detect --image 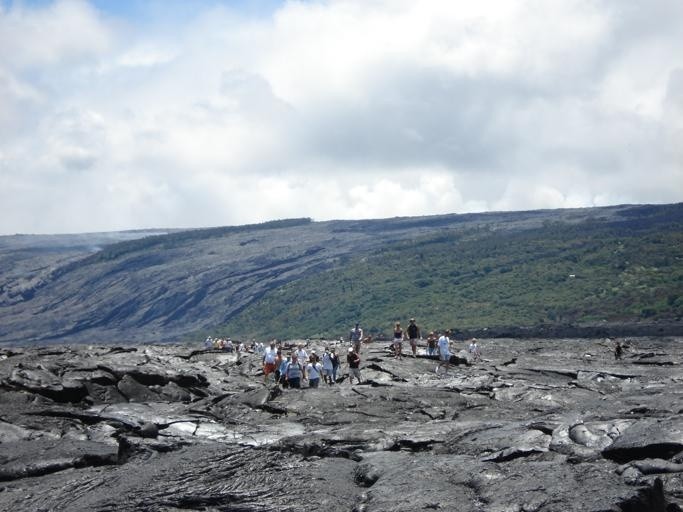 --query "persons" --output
[433,329,451,374]
[425,332,434,358]
[613,341,624,361]
[468,338,478,362]
[434,333,441,354]
[202,323,364,389]
[391,321,404,360]
[405,317,422,357]
